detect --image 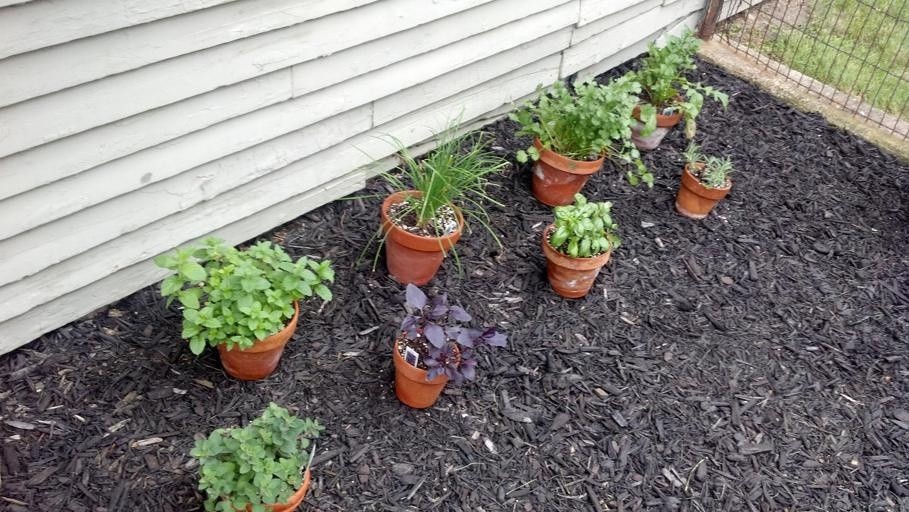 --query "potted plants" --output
[189,401,327,512]
[392,282,509,410]
[511,77,656,207]
[153,236,337,385]
[352,105,507,285]
[675,140,733,222]
[541,191,621,300]
[622,26,730,152]
